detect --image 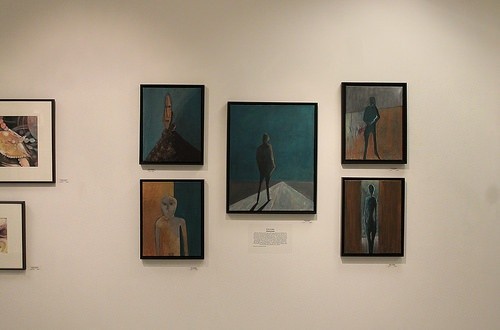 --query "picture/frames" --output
[340,176,405,257]
[139,84,204,166]
[0,201,26,270]
[225,100,317,214]
[0,99,57,184]
[139,178,204,259]
[340,81,408,164]
